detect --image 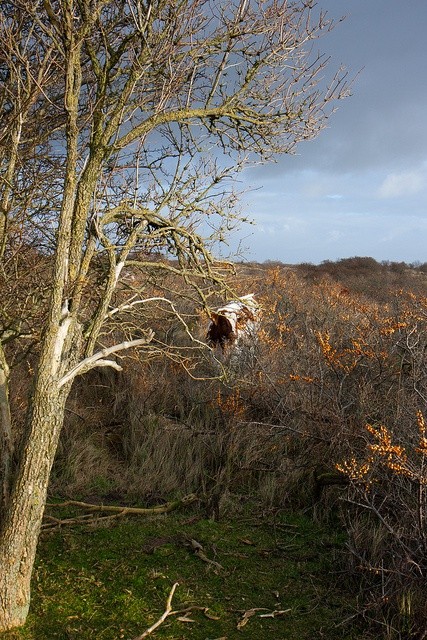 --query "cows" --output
[208,294,266,365]
